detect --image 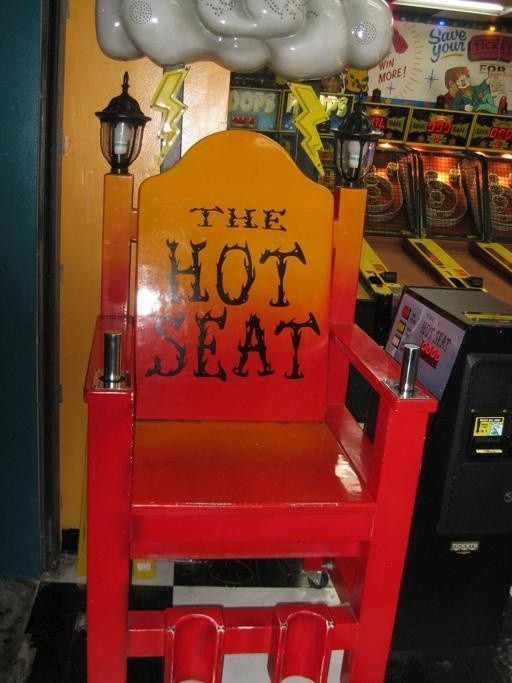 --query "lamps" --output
[94,71,153,176]
[332,106,384,189]
